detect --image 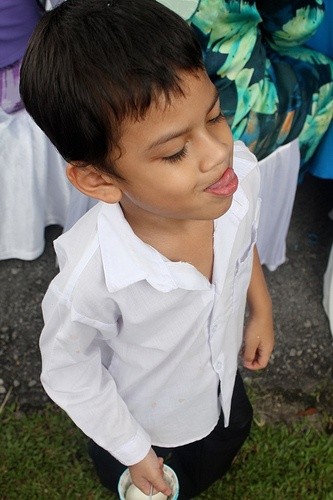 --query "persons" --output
[19,3,274,500]
